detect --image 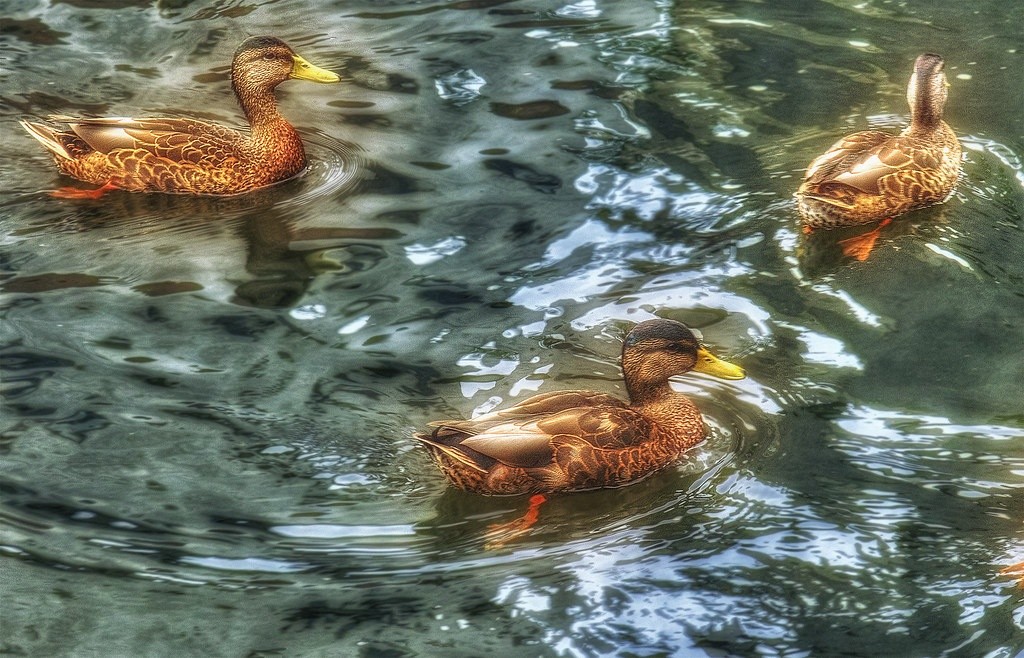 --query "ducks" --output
[19,35,341,199]
[795,52,962,264]
[411,318,747,548]
